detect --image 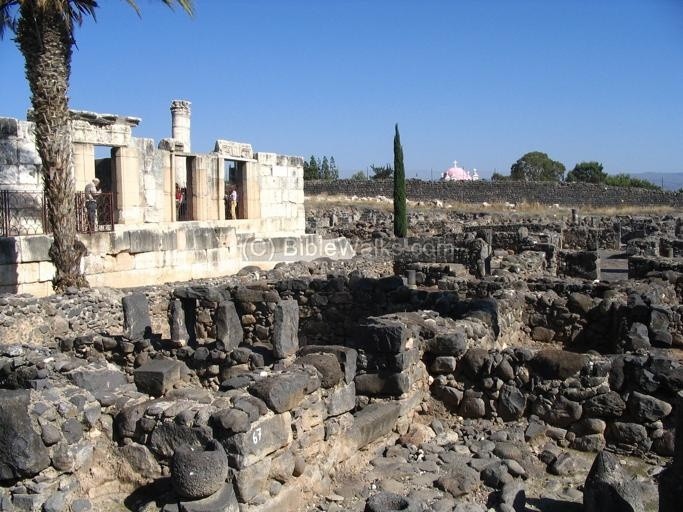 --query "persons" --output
[84,178,102,233]
[176,183,183,220]
[224,184,238,220]
